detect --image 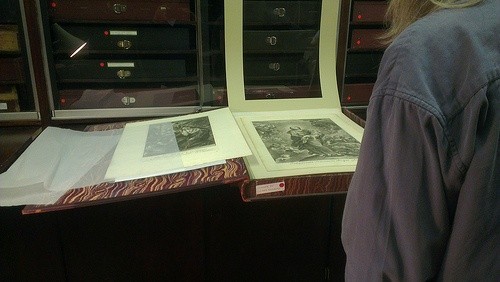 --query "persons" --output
[341,0,500,282]
